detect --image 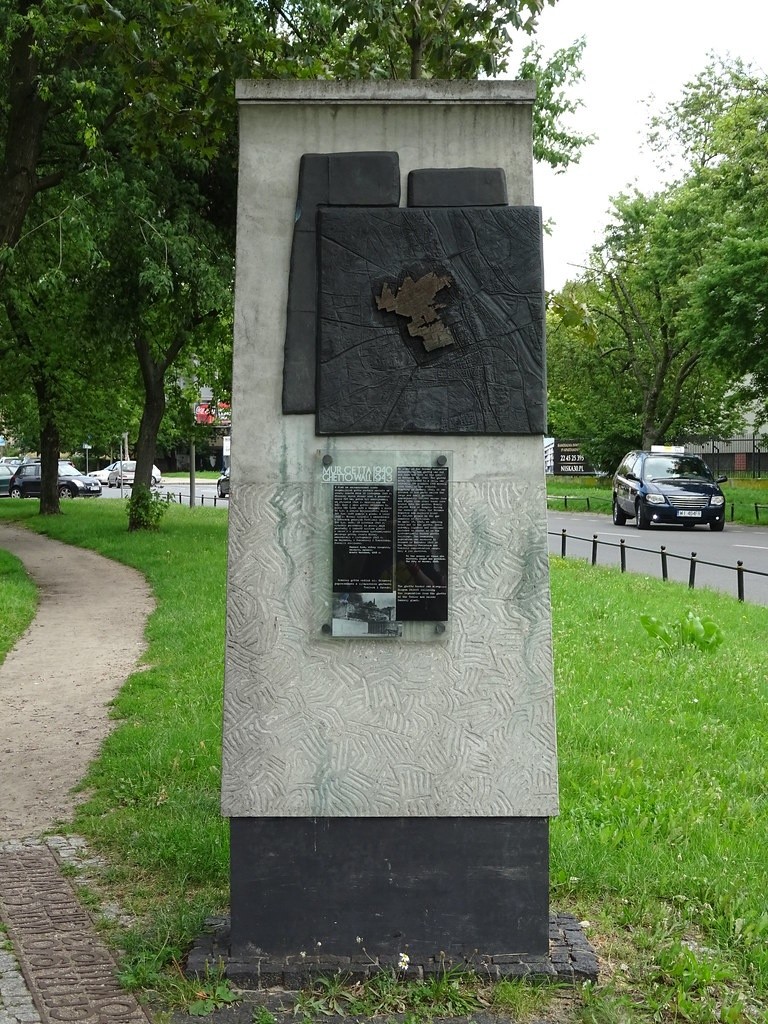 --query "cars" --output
[0,457,102,499]
[88,461,161,486]
[217,466,231,498]
[612,450,728,532]
[107,461,137,488]
